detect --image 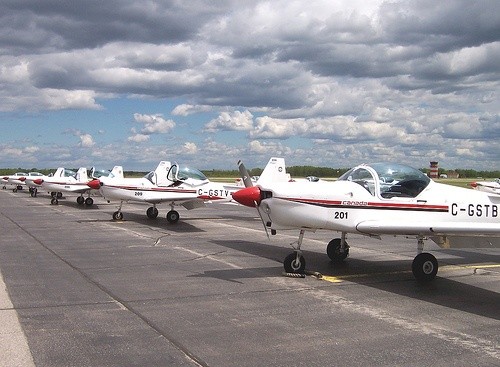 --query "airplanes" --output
[230,152,500,285]
[1,166,117,207]
[88,163,233,225]
